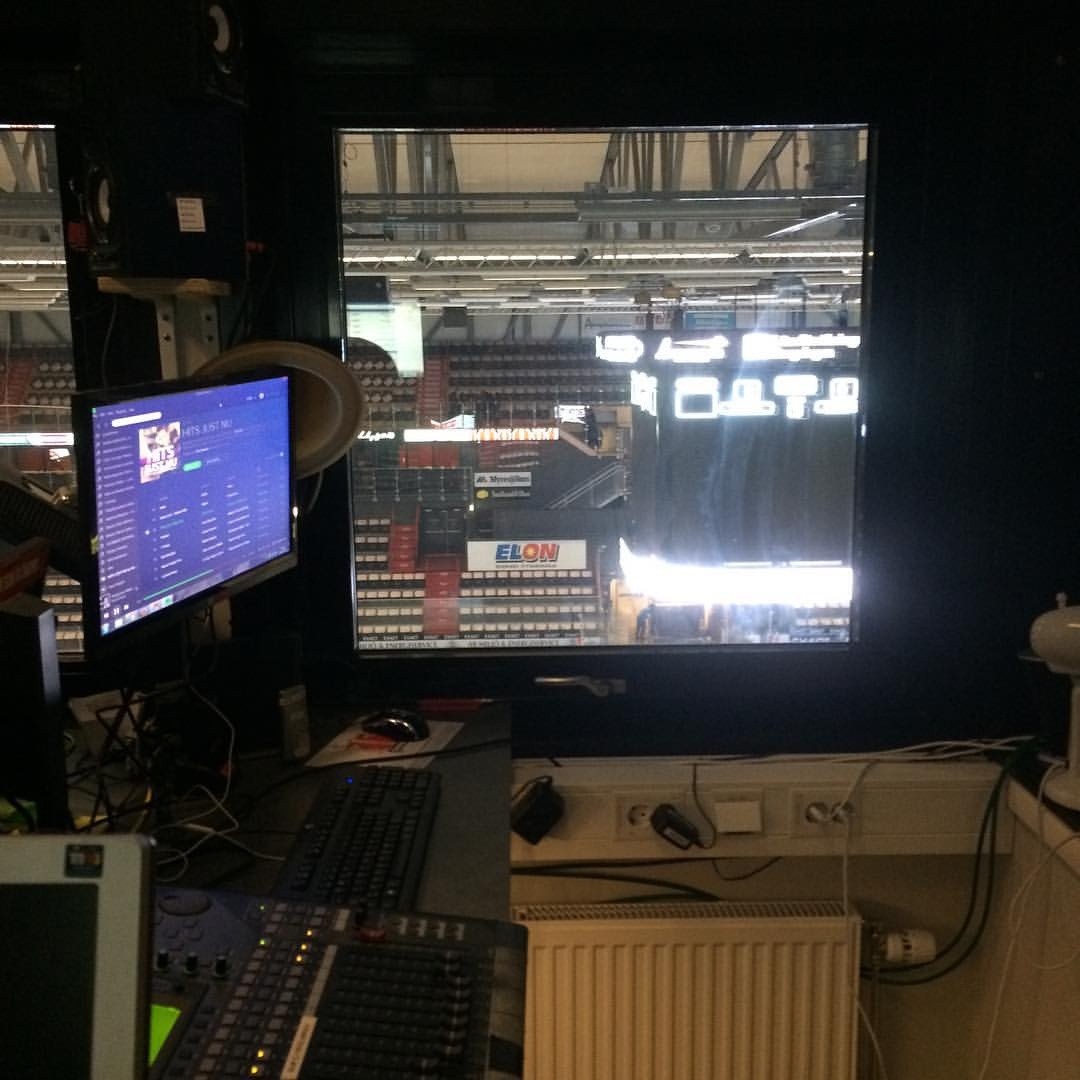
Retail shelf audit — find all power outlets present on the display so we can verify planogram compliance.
[616,791,706,844]
[788,788,863,838]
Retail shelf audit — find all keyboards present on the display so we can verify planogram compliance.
[265,763,443,915]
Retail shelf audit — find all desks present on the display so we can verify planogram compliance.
[1,699,518,1080]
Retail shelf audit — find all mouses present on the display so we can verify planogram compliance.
[360,706,432,744]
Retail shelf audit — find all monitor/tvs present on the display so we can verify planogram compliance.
[1,834,159,1080]
[69,366,302,660]
